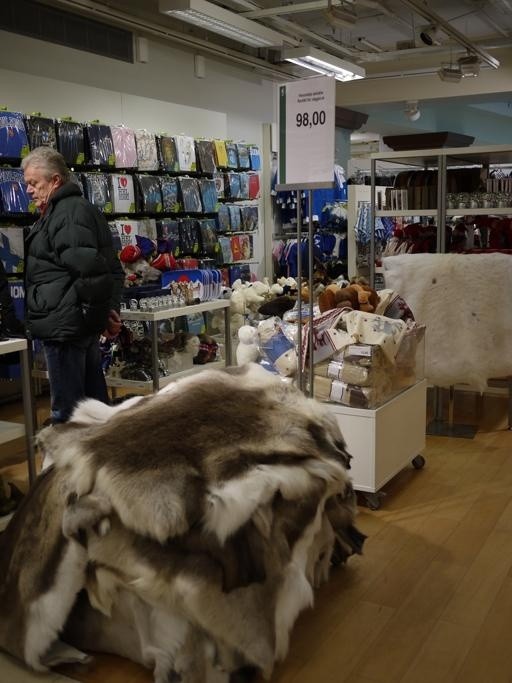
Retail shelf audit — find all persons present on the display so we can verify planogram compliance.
[19,145,123,429]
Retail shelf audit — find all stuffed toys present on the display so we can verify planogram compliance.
[218,275,381,322]
[234,326,259,367]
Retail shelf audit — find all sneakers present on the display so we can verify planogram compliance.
[193,338,217,363]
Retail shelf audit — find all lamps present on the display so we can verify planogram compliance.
[281,46,366,82]
[158,0,284,48]
